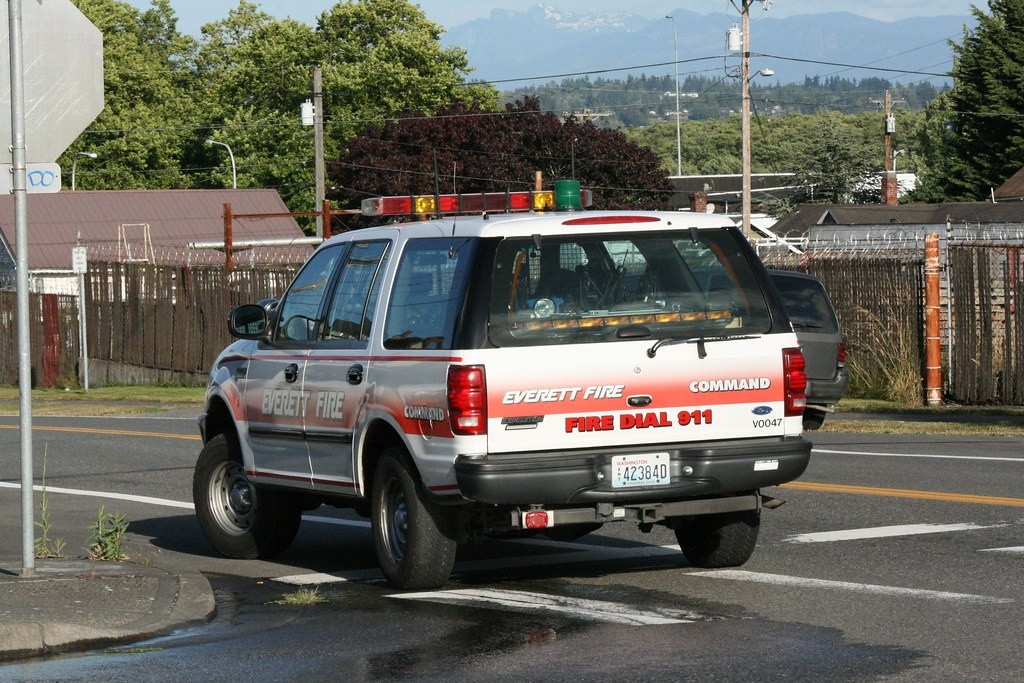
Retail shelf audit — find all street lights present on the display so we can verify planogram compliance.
[205,138,237,189]
[891,148,908,171]
[664,15,682,175]
[739,67,775,240]
[571,138,579,180]
[70,151,98,191]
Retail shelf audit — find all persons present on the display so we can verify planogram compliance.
[544,268,582,313]
[789,285,822,320]
[639,261,673,300]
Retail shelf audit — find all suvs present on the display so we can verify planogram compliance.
[624,266,850,431]
[192,176,814,594]
